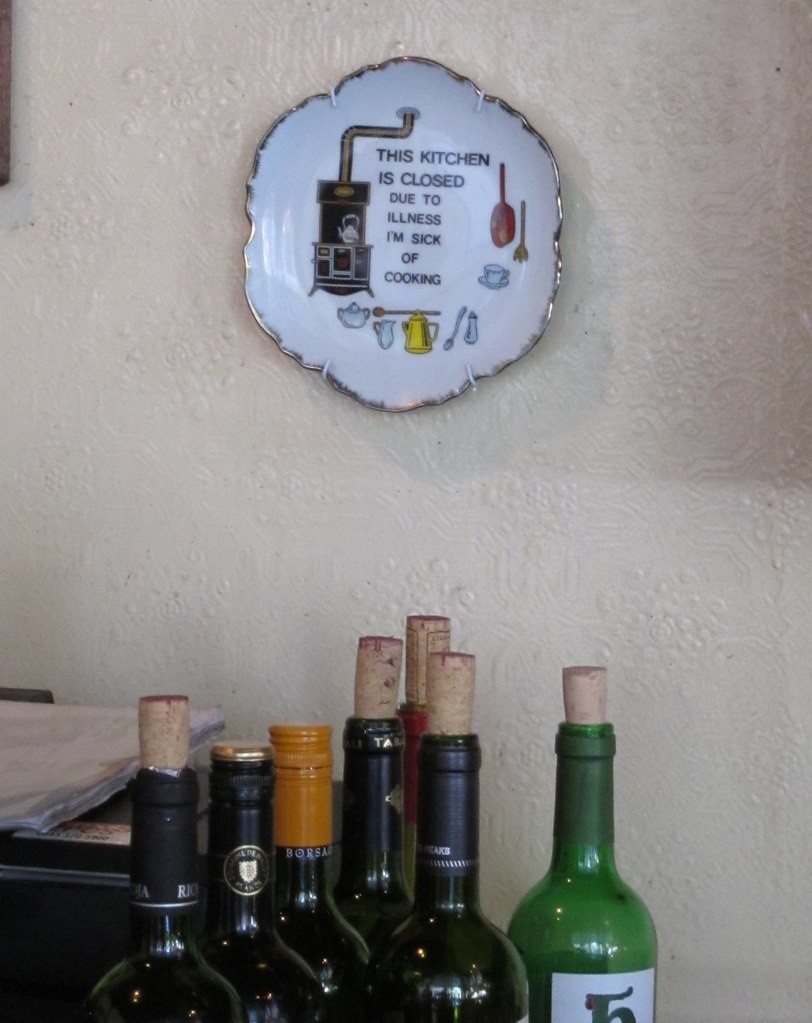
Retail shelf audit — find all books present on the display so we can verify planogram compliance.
[0,698,226,833]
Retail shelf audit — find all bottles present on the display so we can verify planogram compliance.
[83,694,246,1022]
[505,665,660,1022]
[332,636,413,954]
[401,613,454,903]
[267,726,369,1021]
[380,656,529,1022]
[199,742,322,1021]
